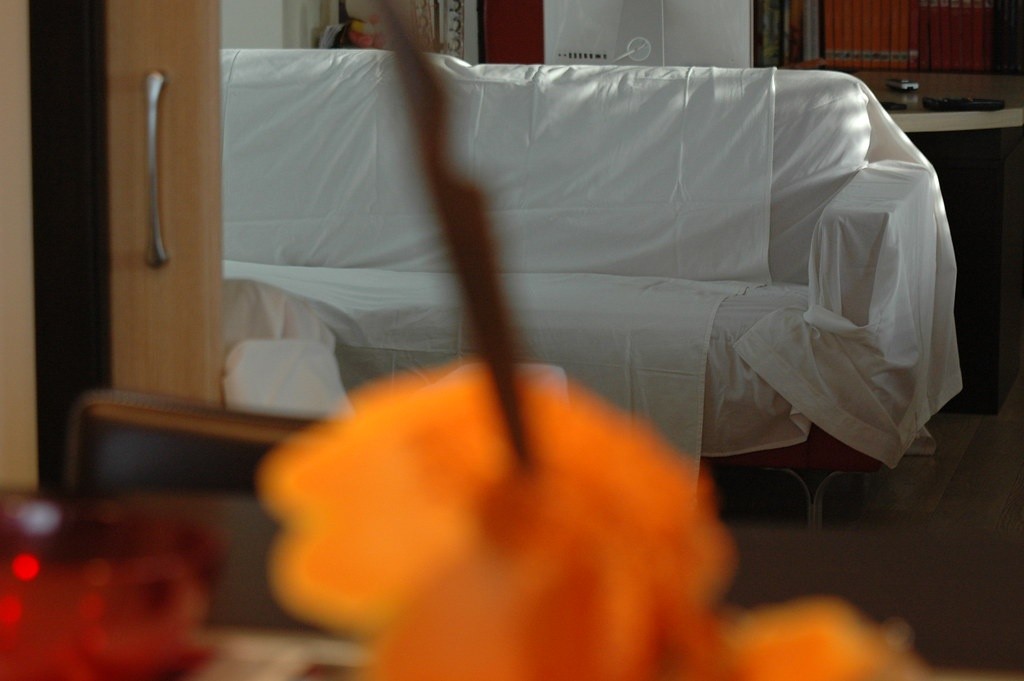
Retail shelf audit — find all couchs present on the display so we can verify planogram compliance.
[218,50,965,529]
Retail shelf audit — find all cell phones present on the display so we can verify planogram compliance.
[885,78,919,91]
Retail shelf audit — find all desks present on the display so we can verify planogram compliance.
[858,72,1024,413]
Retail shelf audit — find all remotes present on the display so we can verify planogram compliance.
[923,97,1005,112]
